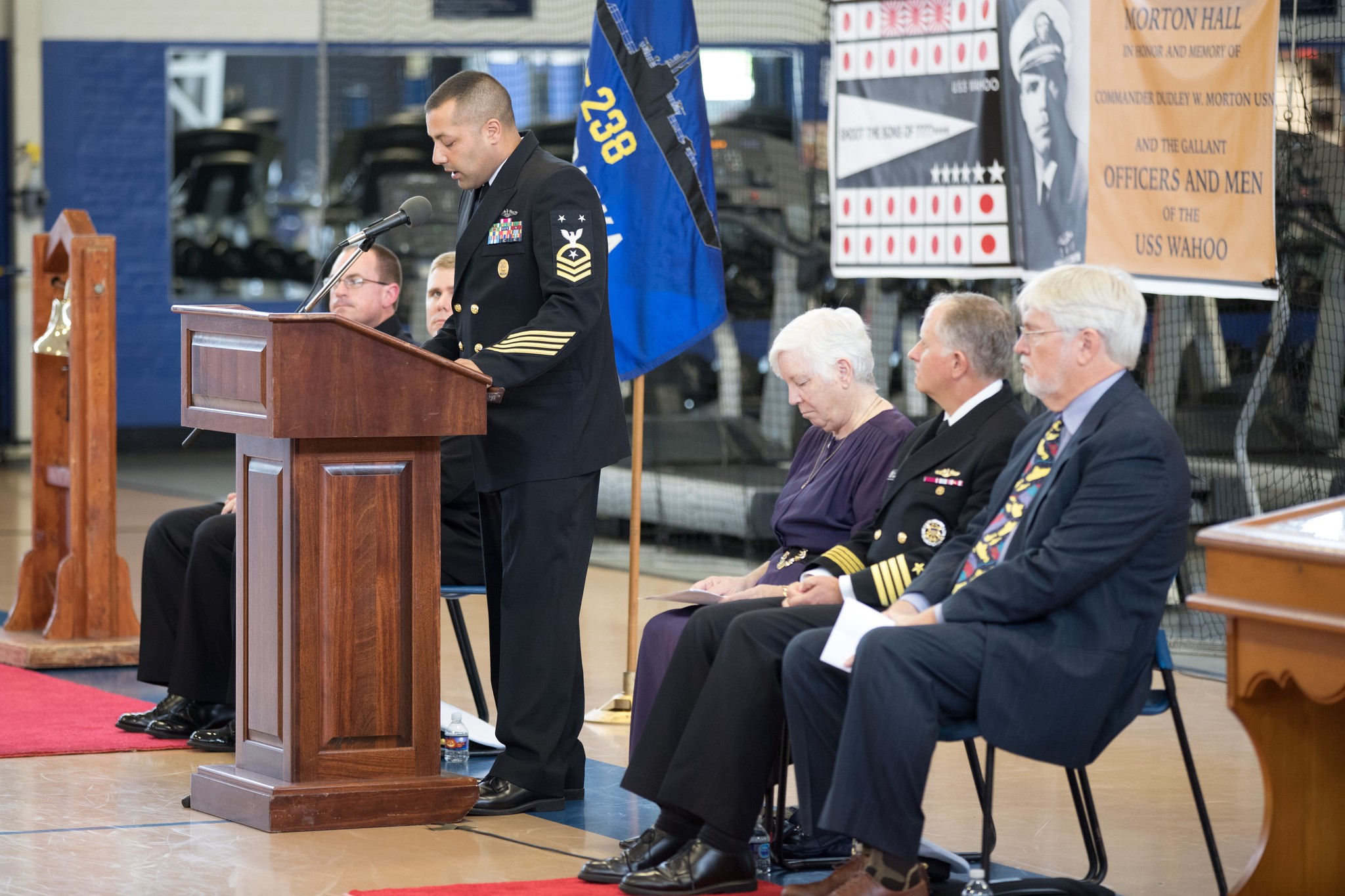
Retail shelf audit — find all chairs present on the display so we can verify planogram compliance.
[438,584,491,723]
[761,629,1233,896]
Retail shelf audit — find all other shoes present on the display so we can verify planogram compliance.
[781,832,852,866]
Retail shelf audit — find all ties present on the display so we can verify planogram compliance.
[478,183,489,203]
[934,421,951,436]
[951,417,1065,594]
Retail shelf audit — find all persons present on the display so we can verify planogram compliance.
[575,289,1030,896]
[113,243,489,752]
[780,263,1193,896]
[413,70,631,816]
[1011,0,1089,273]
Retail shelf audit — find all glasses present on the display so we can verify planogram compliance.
[323,276,391,291]
[1020,325,1093,344]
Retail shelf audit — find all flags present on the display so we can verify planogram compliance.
[571,0,728,382]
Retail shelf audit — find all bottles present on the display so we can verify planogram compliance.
[961,868,993,896]
[748,815,771,874]
[444,712,470,763]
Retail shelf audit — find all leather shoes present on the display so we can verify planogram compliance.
[468,776,566,816]
[188,719,237,750]
[114,693,184,732]
[563,780,584,801]
[144,702,212,739]
[578,825,685,883]
[619,837,757,896]
[781,852,930,896]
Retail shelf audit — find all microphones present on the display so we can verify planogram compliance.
[338,195,433,249]
